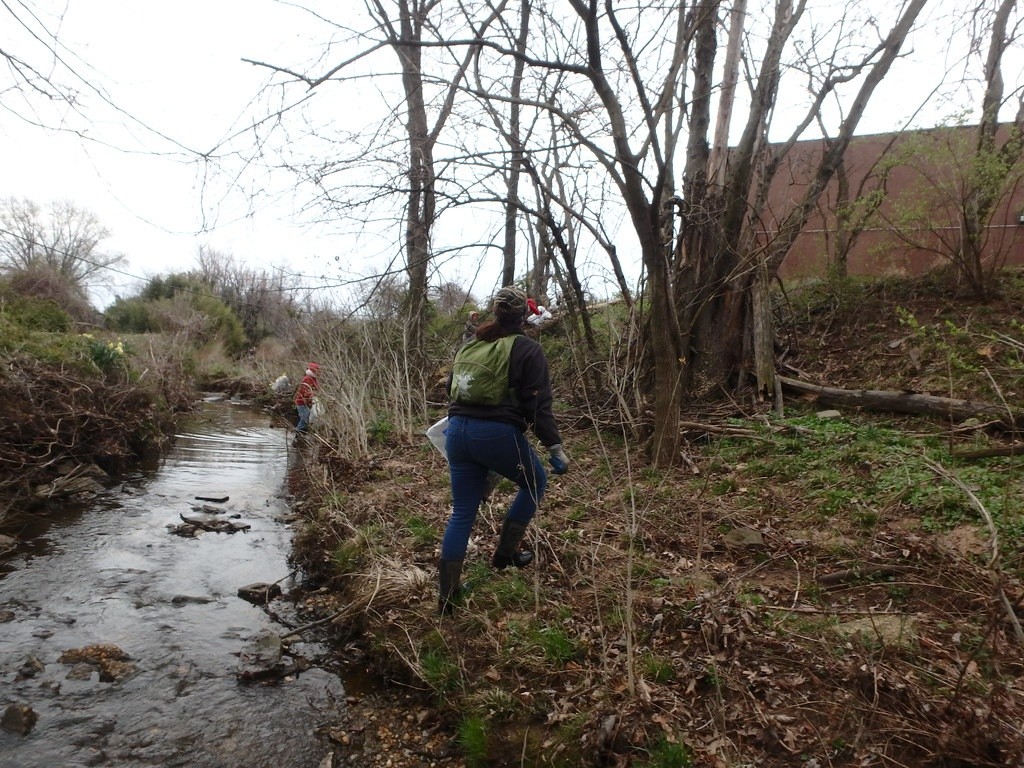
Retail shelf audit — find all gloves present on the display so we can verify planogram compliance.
[548,444,570,475]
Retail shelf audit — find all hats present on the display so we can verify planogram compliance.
[309,362,319,374]
[494,286,528,323]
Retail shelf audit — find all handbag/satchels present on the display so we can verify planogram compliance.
[308,397,325,423]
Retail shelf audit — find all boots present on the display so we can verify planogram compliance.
[292,432,305,447]
[438,558,473,614]
[492,515,532,569]
